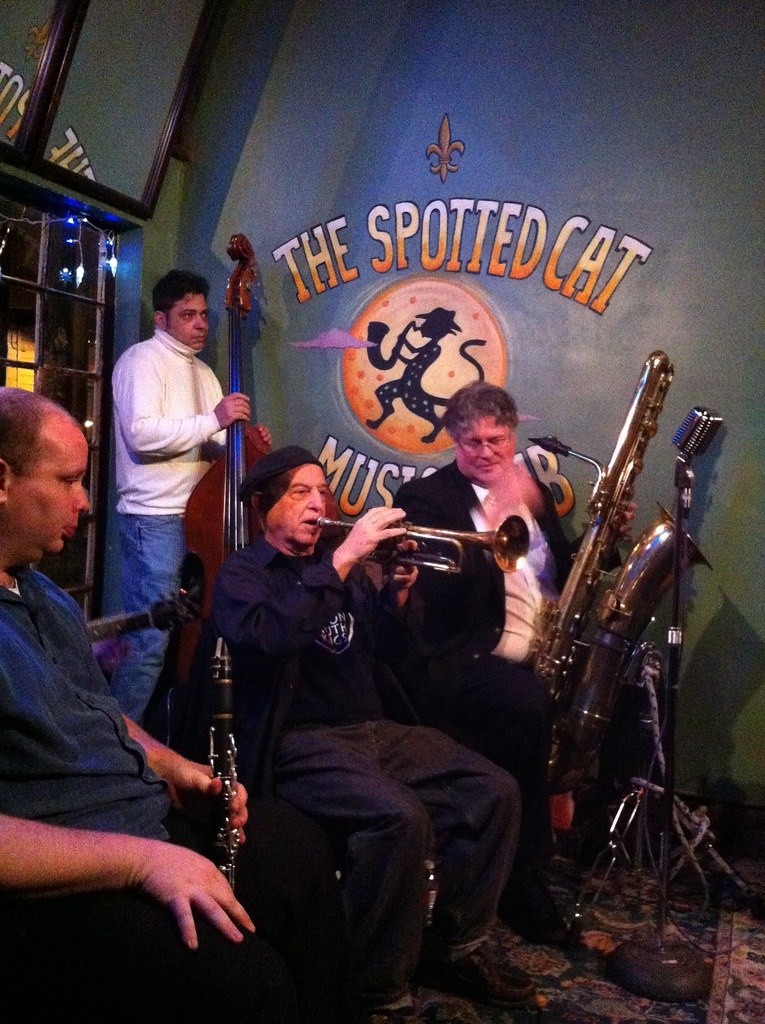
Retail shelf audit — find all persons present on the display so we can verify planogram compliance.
[194,445,536,1024]
[105,269,272,725]
[0,388,360,1024]
[383,381,637,950]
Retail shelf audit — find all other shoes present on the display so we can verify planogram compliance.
[363,990,420,1024]
[498,851,568,942]
[414,927,535,1008]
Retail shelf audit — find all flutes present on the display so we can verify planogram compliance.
[203,633,244,889]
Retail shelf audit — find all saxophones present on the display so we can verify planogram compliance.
[531,347,695,786]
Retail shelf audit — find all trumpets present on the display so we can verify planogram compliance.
[314,512,534,578]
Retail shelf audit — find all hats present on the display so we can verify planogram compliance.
[237,445,323,501]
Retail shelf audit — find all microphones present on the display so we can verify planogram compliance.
[670,405,724,457]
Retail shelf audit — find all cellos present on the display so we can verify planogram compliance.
[169,232,338,700]
